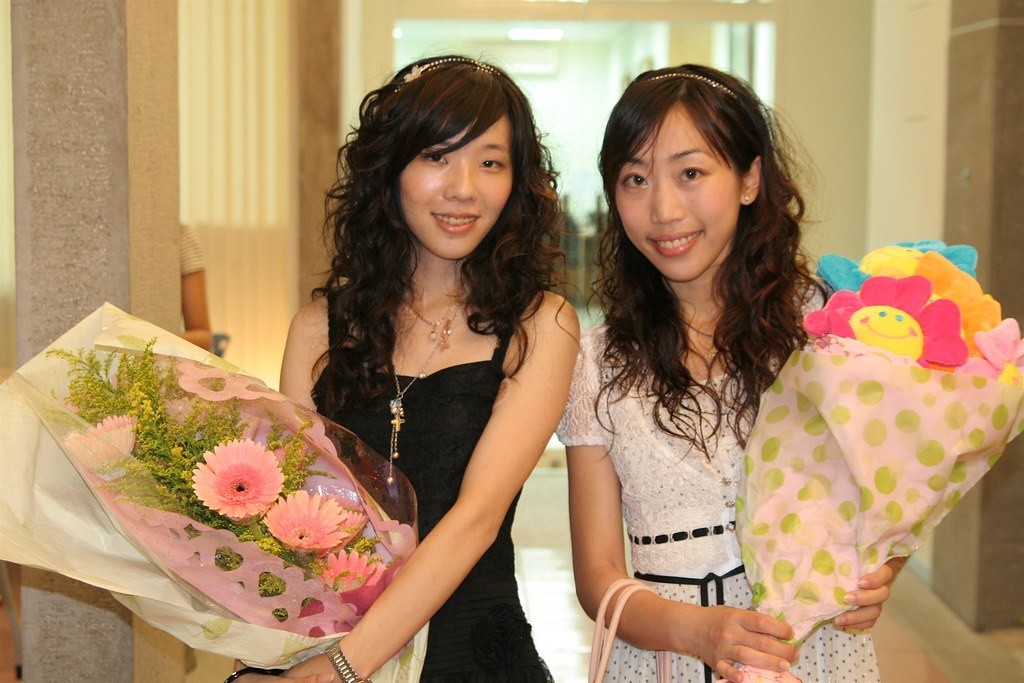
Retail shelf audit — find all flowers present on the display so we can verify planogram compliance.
[0,301,431,683]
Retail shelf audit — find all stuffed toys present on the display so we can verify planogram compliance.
[804,239,1024,375]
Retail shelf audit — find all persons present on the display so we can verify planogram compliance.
[556,63,909,683]
[224,55,581,683]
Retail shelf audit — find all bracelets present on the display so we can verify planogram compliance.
[224,667,269,683]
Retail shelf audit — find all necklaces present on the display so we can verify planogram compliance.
[687,334,720,360]
[396,295,465,341]
[383,302,467,485]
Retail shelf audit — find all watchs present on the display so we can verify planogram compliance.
[325,640,372,683]
